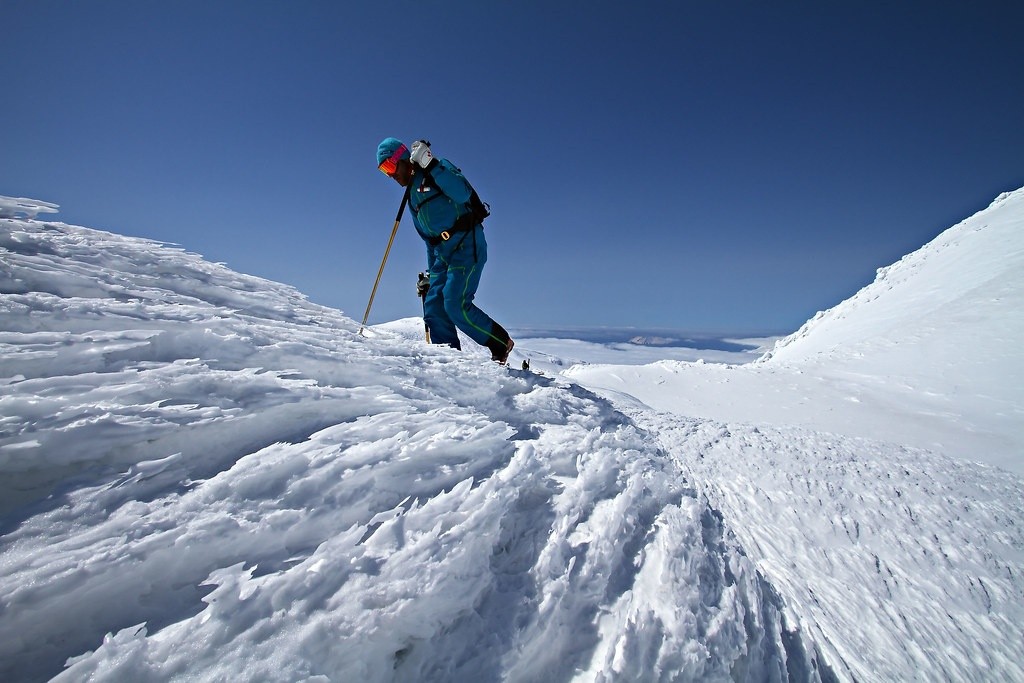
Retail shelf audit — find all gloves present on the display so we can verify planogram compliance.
[409,141,433,168]
[417,273,430,297]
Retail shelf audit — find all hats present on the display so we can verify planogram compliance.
[376,138,410,165]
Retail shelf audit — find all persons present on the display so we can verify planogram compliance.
[377,137,514,364]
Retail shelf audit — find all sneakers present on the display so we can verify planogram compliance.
[492,338,514,364]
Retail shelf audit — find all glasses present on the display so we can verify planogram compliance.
[379,144,407,177]
